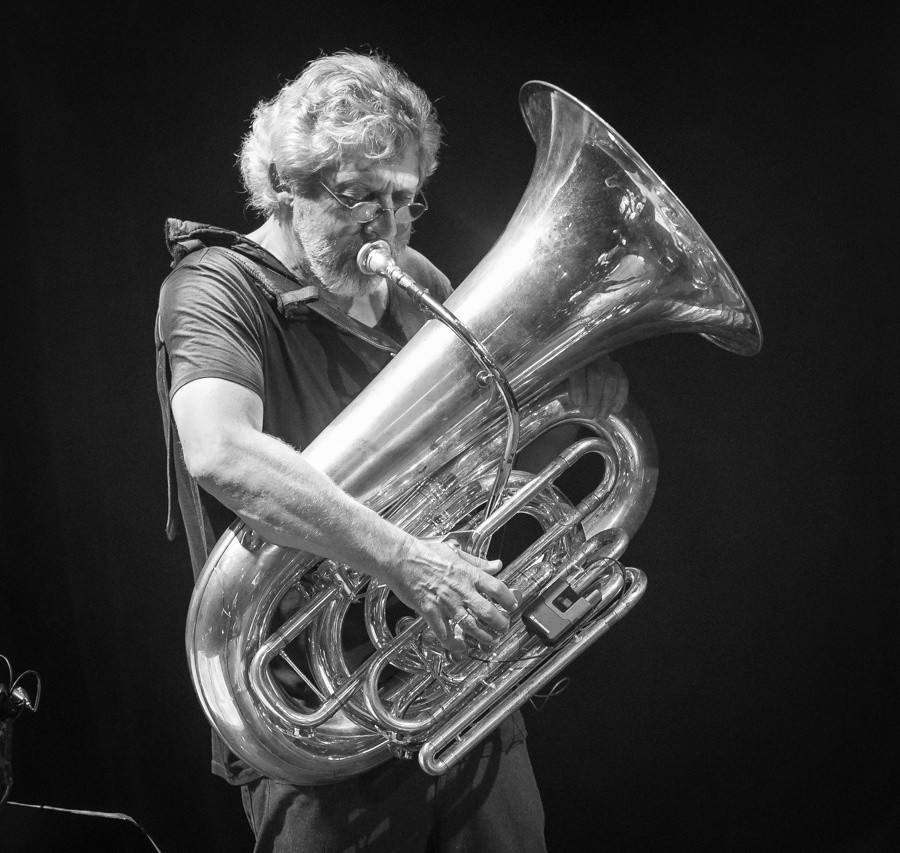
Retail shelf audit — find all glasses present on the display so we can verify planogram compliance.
[316,180,429,224]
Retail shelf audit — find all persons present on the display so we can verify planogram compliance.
[154,52,547,853]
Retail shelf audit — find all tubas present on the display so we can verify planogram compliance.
[183,79,766,787]
